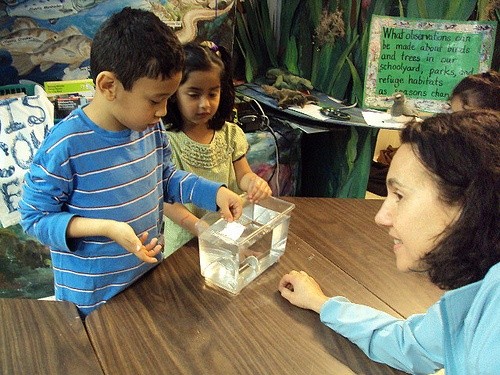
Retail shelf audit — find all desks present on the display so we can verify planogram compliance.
[85,196,448,375]
[0,298,104,375]
[236,83,424,199]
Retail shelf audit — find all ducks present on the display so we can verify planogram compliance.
[381,93,434,123]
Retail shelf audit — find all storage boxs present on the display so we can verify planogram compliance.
[45,79,94,93]
[195,191,296,295]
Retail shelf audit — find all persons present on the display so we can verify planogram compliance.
[449,70,500,113]
[16,7,245,320]
[278,109,500,375]
[161,42,272,261]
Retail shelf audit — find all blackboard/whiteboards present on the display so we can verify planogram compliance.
[362,14,497,118]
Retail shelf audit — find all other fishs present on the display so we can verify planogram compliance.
[205,255,260,276]
